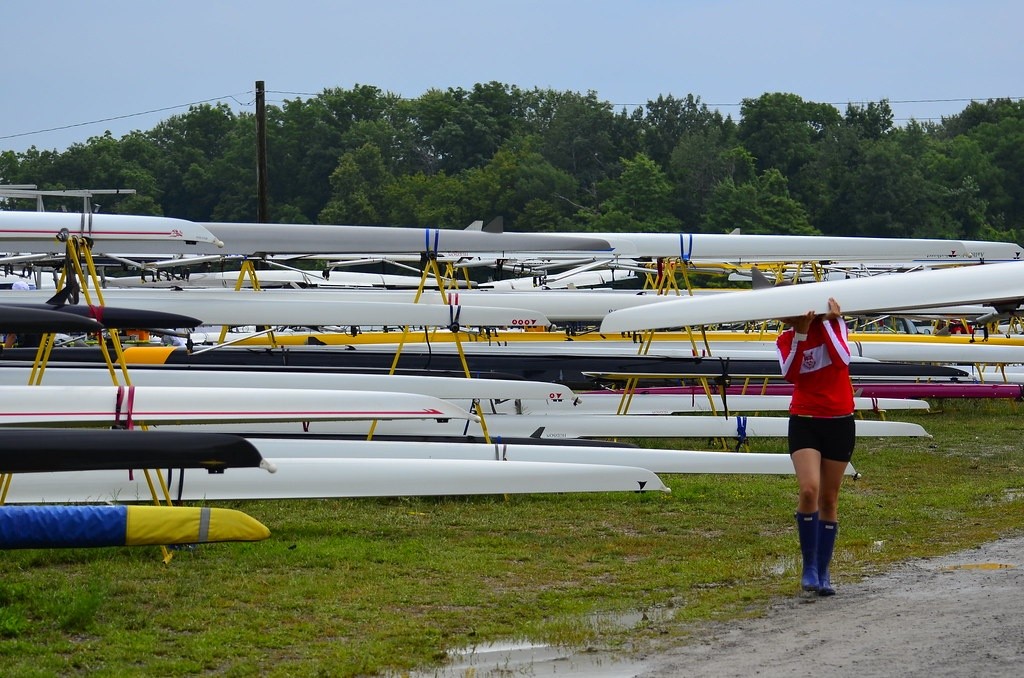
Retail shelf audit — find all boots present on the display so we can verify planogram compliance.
[796,510,820,591]
[819,520,839,596]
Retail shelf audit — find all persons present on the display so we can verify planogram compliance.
[776,297,856,594]
[5,281,43,348]
[151,329,185,346]
[936,320,975,366]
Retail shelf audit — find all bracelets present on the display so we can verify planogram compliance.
[795,332,807,341]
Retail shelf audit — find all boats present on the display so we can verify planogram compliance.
[1,186,1023,546]
